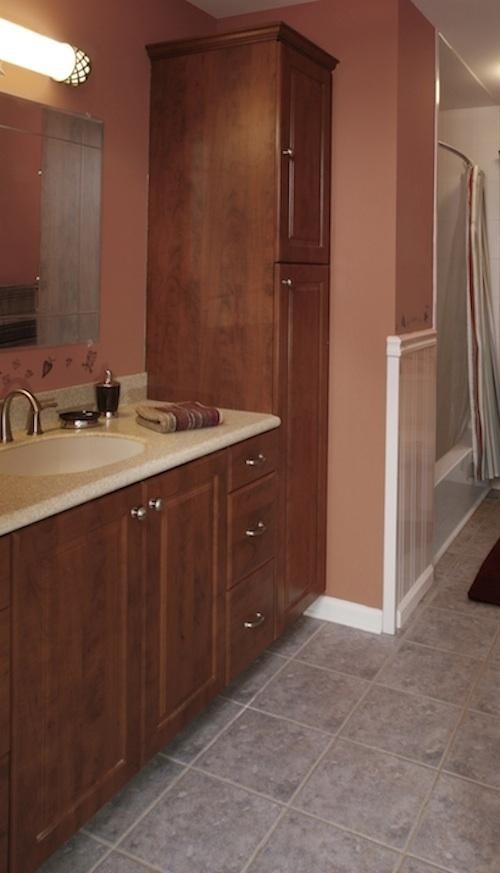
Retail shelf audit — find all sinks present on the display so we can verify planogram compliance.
[0,432,151,476]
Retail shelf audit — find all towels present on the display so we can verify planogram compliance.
[134,395,222,434]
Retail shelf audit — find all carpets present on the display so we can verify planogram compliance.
[467,540,499,606]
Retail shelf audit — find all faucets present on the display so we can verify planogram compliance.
[0,390,44,442]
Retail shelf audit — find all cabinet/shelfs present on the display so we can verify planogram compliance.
[2,450,232,870]
[139,22,335,626]
[212,427,281,694]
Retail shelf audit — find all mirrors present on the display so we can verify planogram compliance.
[1,94,104,350]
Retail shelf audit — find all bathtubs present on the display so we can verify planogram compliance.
[432,426,473,487]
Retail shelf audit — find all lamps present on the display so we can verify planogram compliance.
[1,18,92,88]
